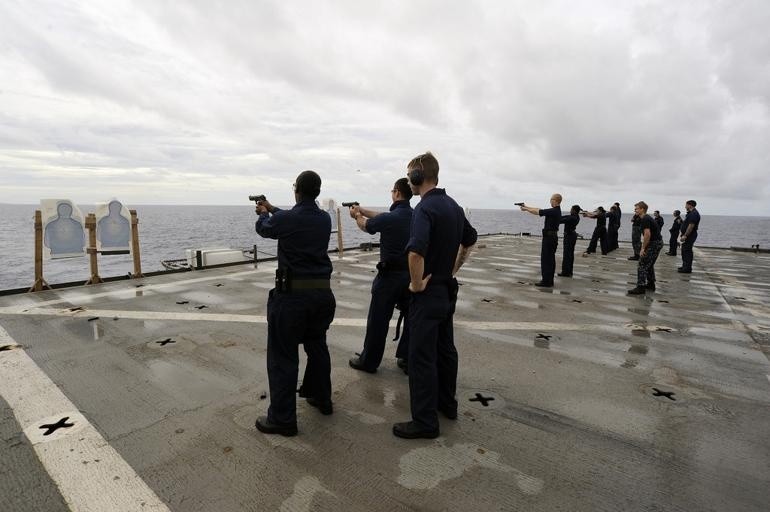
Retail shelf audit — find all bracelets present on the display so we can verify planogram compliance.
[355,212,361,220]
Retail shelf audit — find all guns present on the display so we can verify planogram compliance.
[515,203,524,206]
[342,202,359,207]
[249,195,266,204]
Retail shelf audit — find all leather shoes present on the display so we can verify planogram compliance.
[628,287,645,295]
[398,357,409,376]
[349,358,377,373]
[665,252,677,256]
[678,267,692,273]
[645,284,655,291]
[557,272,573,276]
[535,282,554,287]
[305,398,332,415]
[438,407,458,421]
[255,416,298,436]
[628,256,639,260]
[393,421,440,439]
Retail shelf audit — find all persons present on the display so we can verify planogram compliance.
[393,155,478,438]
[350,178,414,372]
[255,171,335,436]
[522,195,701,296]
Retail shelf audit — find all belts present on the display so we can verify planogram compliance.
[290,279,330,288]
